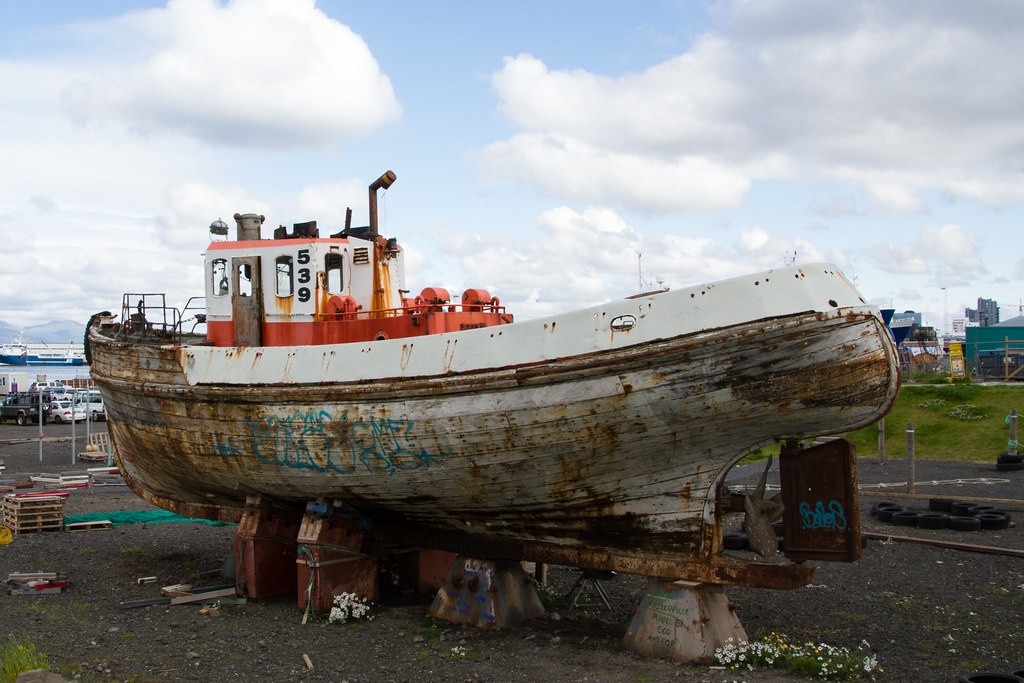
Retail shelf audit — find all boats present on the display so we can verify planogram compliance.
[84,170,902,589]
[0,331,87,366]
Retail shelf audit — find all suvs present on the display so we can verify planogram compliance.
[0,394,53,426]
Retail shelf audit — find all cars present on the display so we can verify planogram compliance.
[29,382,108,424]
